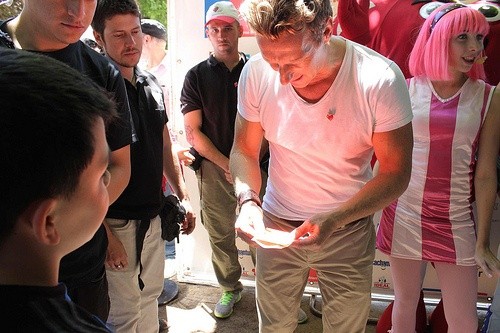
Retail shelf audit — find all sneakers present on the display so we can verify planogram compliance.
[214,290,241,318]
[298,309,307,323]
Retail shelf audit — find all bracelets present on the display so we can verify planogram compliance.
[239,190,261,209]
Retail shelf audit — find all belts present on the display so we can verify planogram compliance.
[284,217,366,232]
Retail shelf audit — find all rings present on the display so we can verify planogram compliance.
[116,264,122,268]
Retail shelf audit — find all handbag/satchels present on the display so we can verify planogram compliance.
[159,194,186,243]
[187,146,203,172]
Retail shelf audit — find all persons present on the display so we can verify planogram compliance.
[0,0,195,333]
[229,0,413,333]
[180,0,307,325]
[378,2,500,333]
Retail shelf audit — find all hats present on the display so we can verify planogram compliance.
[205,1,241,27]
[141,19,167,41]
